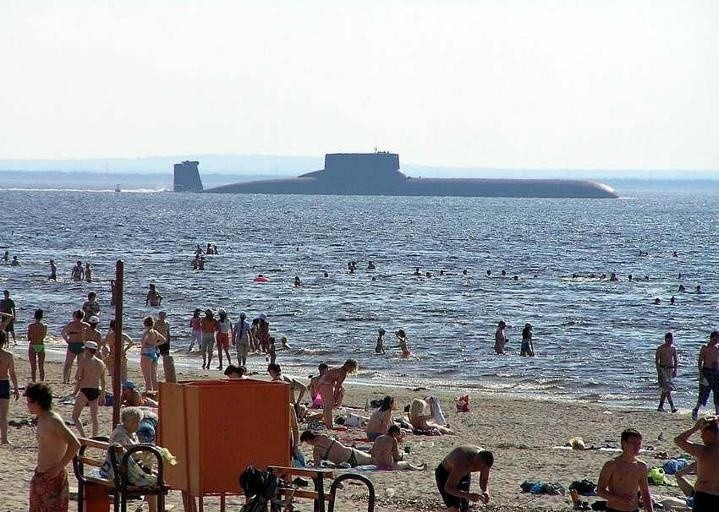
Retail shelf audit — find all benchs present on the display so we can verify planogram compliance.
[70,433,170,512]
[261,462,377,511]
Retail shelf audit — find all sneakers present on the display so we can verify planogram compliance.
[657,407,668,412]
[692,408,697,421]
[672,408,677,412]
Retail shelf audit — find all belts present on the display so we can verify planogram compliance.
[660,364,675,369]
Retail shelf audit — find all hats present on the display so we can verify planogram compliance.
[258,313,267,320]
[218,308,226,314]
[205,310,212,315]
[81,340,97,349]
[240,312,246,318]
[280,336,287,343]
[88,315,99,323]
[525,323,534,328]
